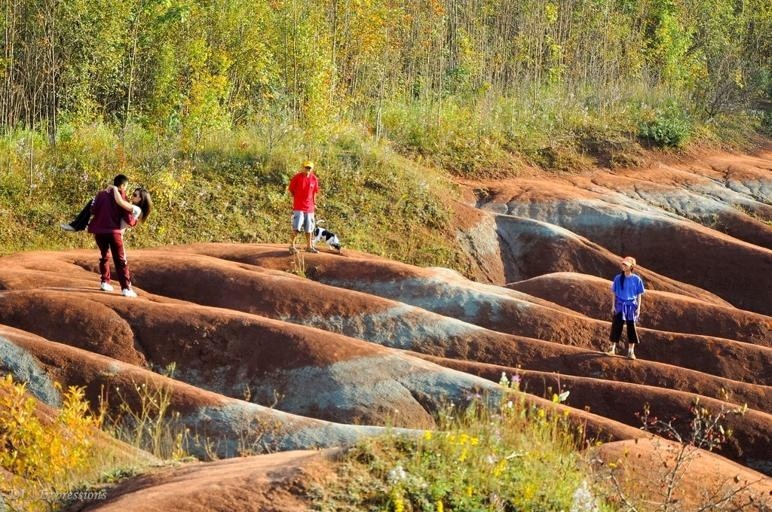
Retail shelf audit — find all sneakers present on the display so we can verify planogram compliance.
[60,224,76,230]
[288,245,319,255]
[101,282,114,291]
[122,288,138,297]
[605,350,636,360]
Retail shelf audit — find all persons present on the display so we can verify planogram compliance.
[289,161,319,255]
[60,175,152,297]
[604,256,642,360]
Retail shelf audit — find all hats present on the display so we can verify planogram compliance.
[621,256,638,268]
[304,160,315,169]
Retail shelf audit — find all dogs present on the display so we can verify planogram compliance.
[311,220,343,254]
